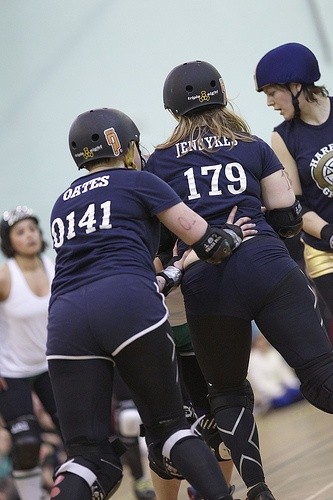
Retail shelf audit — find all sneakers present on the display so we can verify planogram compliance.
[133,479,156,500]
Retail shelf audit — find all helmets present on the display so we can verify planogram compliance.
[163,60,227,117]
[0,207,39,257]
[255,42,322,92]
[68,107,140,170]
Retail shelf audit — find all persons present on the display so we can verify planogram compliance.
[252,43,333,340]
[142,60,333,500]
[0,206,302,500]
[44,108,258,500]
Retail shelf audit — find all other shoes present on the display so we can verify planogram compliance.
[246,483,277,500]
